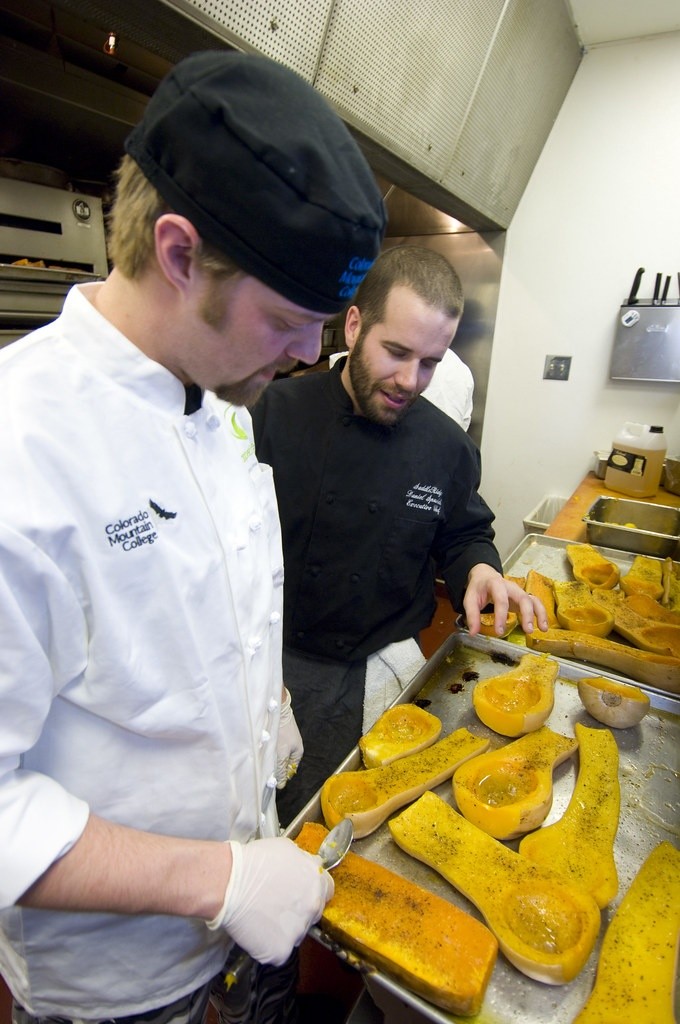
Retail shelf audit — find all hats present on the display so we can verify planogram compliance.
[124,51,386,315]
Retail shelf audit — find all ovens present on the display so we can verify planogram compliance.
[0,176,108,351]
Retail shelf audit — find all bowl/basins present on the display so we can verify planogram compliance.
[664,458,680,497]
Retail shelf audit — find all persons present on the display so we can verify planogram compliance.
[252,245,548,830]
[0,48,389,1024]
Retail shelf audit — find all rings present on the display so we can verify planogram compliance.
[528,593,532,597]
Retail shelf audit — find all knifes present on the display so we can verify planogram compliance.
[626,267,680,306]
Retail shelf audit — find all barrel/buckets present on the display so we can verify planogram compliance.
[604,422,668,498]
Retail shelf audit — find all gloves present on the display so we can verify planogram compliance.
[207,835,338,967]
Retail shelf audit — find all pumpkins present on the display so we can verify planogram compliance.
[292,542,680,1024]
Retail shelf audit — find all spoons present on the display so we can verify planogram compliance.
[227,817,355,984]
